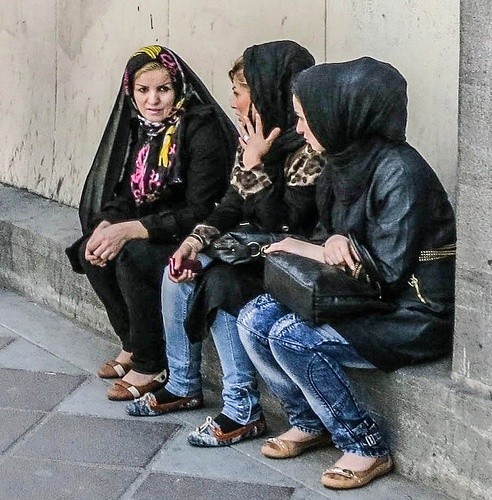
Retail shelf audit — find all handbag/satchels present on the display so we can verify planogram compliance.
[193,219,293,266]
[264,234,400,328]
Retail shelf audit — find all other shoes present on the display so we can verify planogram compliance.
[321,447,392,486]
[107,369,167,401]
[99,359,130,378]
[127,392,202,414]
[186,416,266,446]
[262,421,325,458]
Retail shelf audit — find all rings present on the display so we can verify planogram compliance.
[242,134,249,142]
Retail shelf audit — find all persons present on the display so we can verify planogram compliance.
[125,40,316,448]
[235,56,457,490]
[64,45,239,401]
